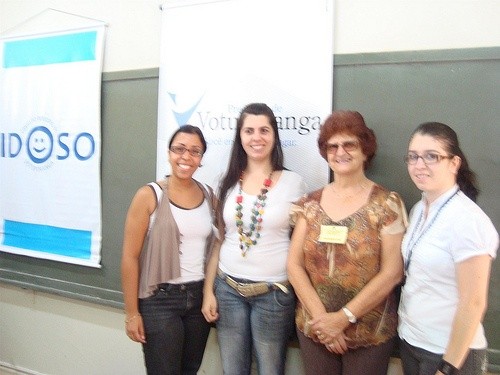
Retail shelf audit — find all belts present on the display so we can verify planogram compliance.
[158,279,204,294]
[217,267,292,298]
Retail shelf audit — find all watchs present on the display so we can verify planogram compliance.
[342,305,358,324]
[438,359,458,375]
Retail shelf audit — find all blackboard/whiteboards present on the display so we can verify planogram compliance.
[0,46,500,375]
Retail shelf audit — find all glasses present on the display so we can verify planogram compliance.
[323,141,361,154]
[169,145,203,157]
[405,151,452,165]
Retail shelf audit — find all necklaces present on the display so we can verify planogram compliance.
[405,187,459,270]
[233,169,275,257]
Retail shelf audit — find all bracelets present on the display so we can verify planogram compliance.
[125,312,140,324]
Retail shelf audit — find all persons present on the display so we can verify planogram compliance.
[200,103,310,375]
[394,121,500,375]
[121,125,219,375]
[285,109,409,375]
